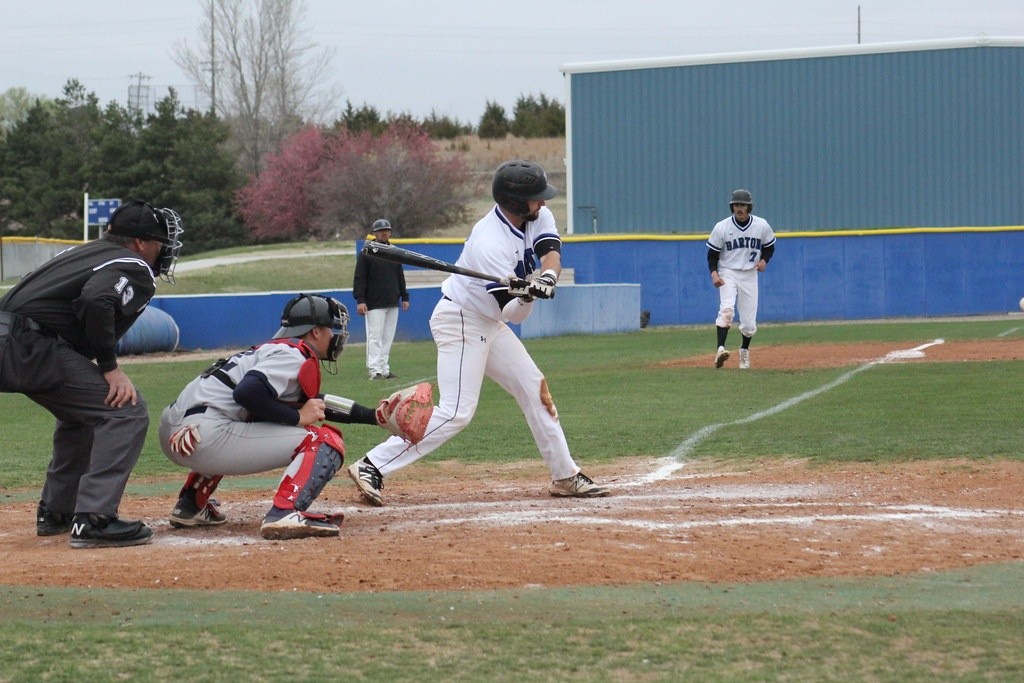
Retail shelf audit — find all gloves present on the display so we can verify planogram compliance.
[169,425,201,458]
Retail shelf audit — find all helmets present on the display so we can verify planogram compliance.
[729,189,753,214]
[492,158,557,215]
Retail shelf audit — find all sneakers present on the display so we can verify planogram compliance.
[169,499,228,527]
[260,507,339,541]
[547,473,610,499]
[69,513,155,549]
[36,500,74,536]
[348,456,385,506]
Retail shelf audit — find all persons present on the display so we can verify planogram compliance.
[347,158,612,506]
[705,189,776,370]
[0,197,185,550]
[157,293,434,541]
[352,219,410,380]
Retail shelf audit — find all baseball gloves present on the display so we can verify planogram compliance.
[376,382,436,443]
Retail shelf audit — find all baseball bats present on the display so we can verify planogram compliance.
[361,239,557,299]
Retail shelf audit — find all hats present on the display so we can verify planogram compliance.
[107,200,182,245]
[372,219,392,232]
[272,293,343,340]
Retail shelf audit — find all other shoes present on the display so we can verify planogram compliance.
[714,350,730,368]
[739,344,750,369]
